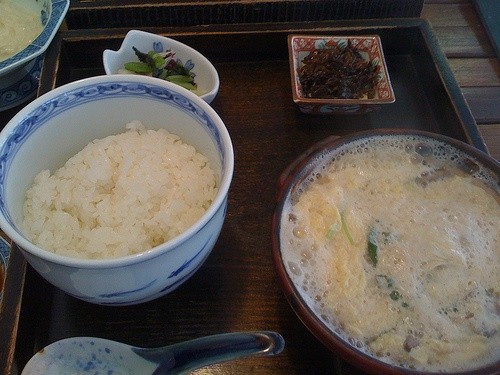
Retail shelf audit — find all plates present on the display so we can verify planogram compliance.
[103,29,220,111]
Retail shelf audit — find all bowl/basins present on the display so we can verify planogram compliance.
[1,74,234,307]
[0,0,70,88]
[273,126,500,375]
[288,32,396,114]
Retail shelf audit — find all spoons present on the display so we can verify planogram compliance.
[24,327,286,375]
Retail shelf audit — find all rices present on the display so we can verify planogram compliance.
[19,119,214,259]
